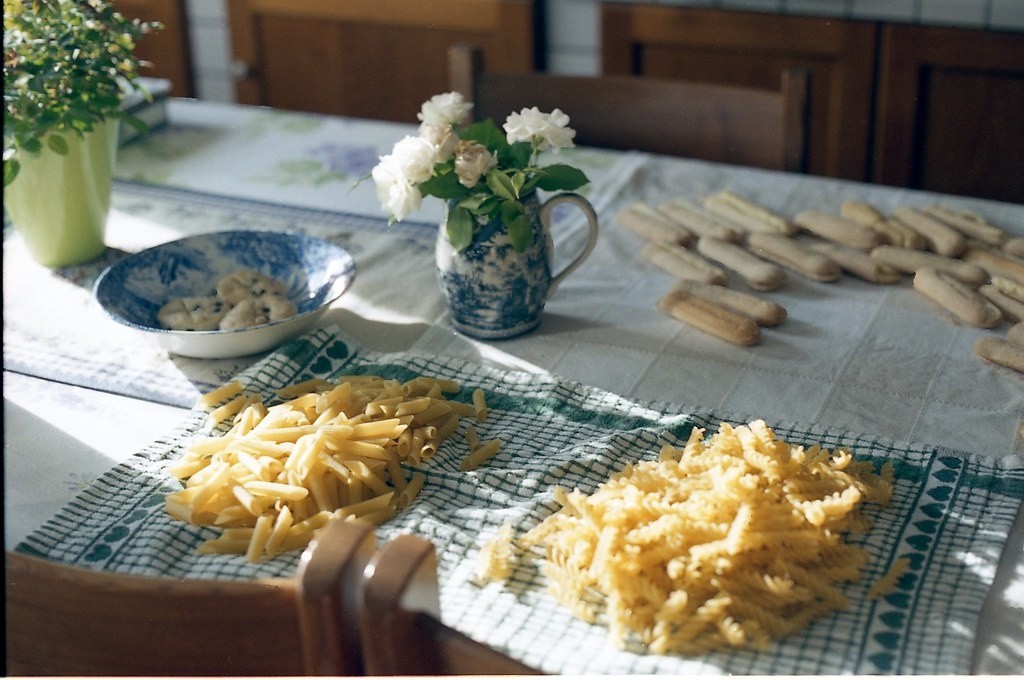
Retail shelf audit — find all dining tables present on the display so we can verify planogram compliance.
[2,96,1024,676]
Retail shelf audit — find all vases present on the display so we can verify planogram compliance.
[436,194,599,339]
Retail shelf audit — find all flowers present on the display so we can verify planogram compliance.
[370,93,593,252]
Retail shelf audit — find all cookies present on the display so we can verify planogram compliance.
[618,193,1024,368]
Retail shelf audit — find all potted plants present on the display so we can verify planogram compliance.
[1,0,167,270]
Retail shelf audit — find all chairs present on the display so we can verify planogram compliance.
[447,40,814,174]
[5,522,544,675]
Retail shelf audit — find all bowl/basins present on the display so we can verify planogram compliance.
[91,227,359,359]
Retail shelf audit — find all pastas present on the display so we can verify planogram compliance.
[161,375,500,565]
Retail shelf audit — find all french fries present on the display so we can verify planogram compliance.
[489,421,909,652]
